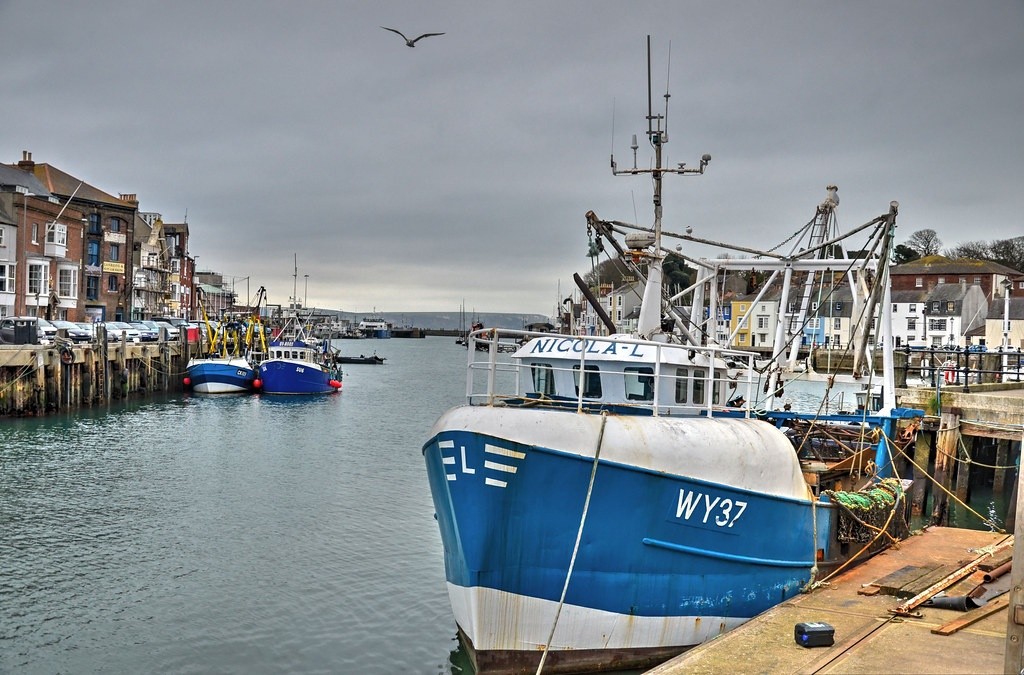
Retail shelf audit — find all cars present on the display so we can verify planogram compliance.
[0,314,272,346]
[968,345,988,352]
[994,345,1016,353]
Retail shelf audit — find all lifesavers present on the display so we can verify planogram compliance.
[944,364,956,384]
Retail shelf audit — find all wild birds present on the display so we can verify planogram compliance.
[379,26,446,48]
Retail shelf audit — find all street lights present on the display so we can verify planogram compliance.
[1000,280,1012,386]
[950,317,955,351]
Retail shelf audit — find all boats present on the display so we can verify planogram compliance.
[418,30,916,675]
[183,286,258,396]
[455,298,516,352]
[329,352,384,365]
[258,253,343,394]
